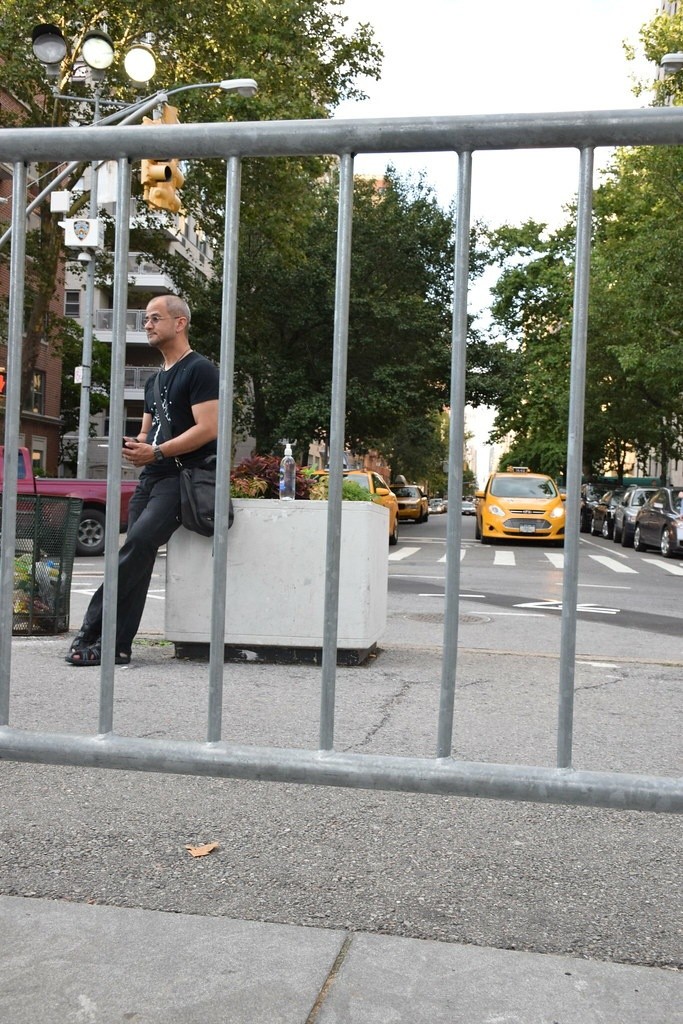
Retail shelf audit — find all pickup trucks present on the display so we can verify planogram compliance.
[0,446,139,558]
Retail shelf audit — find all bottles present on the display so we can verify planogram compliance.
[279,444,296,500]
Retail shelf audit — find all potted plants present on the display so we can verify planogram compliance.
[166,451,390,667]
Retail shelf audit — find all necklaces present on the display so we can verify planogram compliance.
[159,349,190,372]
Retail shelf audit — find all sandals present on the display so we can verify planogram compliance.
[66,642,131,665]
[70,618,100,653]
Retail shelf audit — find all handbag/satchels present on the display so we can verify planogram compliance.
[178,468,235,538]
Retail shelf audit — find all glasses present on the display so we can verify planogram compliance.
[143,316,179,325]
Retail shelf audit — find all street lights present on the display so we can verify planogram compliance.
[72,78,258,472]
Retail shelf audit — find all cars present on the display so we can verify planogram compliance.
[475,466,566,548]
[580,482,683,559]
[559,489,567,501]
[388,483,479,524]
[309,468,400,545]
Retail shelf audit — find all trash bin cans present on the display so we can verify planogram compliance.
[0,493,84,636]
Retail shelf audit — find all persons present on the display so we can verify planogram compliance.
[65,294,220,664]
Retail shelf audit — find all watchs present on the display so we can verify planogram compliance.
[154,445,164,461]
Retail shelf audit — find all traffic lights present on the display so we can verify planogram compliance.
[140,115,172,188]
[142,105,185,216]
